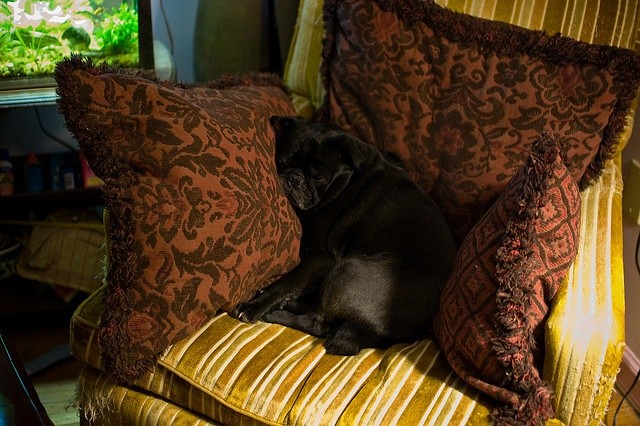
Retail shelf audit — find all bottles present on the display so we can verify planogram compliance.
[0,147,14,196]
[60,167,81,192]
[78,149,106,191]
[24,161,44,192]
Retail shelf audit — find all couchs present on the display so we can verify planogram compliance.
[68,1,640,426]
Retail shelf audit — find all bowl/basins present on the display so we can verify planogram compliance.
[0,235,25,279]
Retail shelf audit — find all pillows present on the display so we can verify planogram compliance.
[316,0,639,224]
[435,139,582,425]
[53,52,302,379]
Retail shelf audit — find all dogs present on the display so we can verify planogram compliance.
[227,115,448,354]
